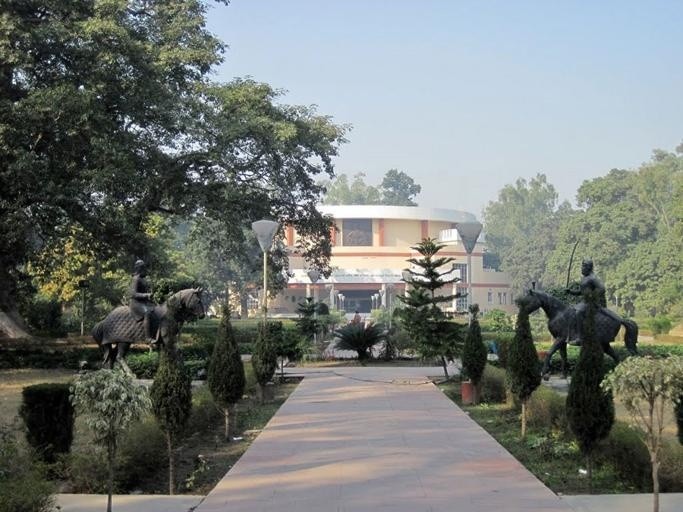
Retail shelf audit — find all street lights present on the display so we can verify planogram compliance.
[401,269,413,303]
[251,218,278,325]
[326,282,385,313]
[307,268,320,344]
[453,220,483,322]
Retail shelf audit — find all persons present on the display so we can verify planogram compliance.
[129,259,158,344]
[353,310,360,326]
[561,256,604,344]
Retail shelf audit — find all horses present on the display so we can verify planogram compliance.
[522,281,643,380]
[91,286,205,379]
